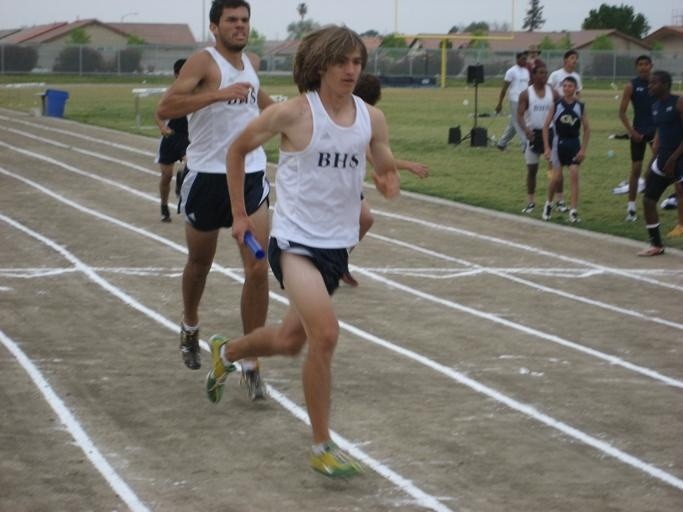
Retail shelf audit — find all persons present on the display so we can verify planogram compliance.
[154,59,189,223]
[619,56,656,221]
[205,23,403,481]
[637,70,683,257]
[341,75,428,287]
[157,1,277,402]
[494,49,590,223]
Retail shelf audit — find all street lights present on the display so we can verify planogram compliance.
[117,9,142,79]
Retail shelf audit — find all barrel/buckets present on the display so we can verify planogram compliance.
[46,88,69,118]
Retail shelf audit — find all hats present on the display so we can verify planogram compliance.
[523,44,542,56]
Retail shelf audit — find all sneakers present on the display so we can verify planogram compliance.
[624,208,639,223]
[554,199,569,214]
[339,265,359,287]
[541,200,554,222]
[239,355,269,403]
[666,223,683,239]
[567,208,582,223]
[306,438,366,483]
[179,312,202,372]
[637,246,665,257]
[521,202,536,215]
[159,204,171,223]
[204,333,236,405]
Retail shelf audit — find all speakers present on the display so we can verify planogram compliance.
[467,64,485,84]
